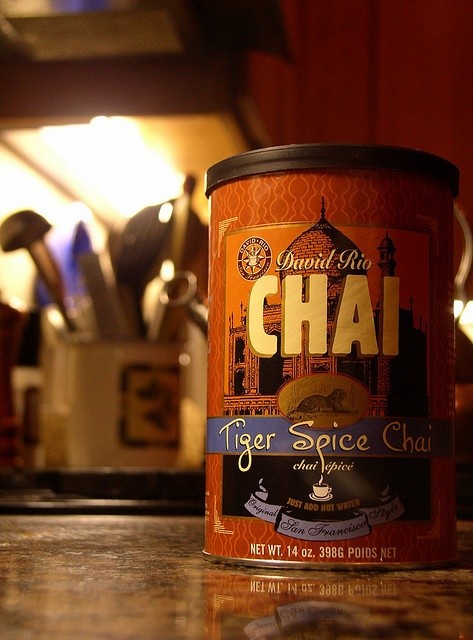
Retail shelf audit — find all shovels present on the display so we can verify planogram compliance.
[113,202,181,336]
